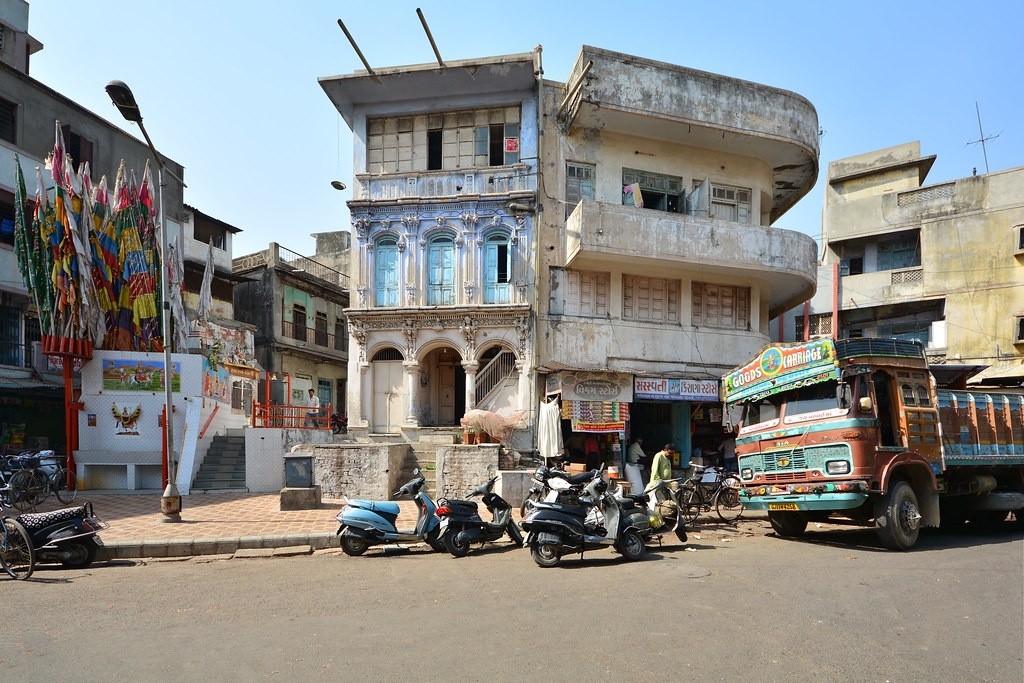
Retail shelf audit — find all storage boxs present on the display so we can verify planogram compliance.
[563,463,587,475]
[2,434,50,455]
[670,452,680,466]
[691,456,704,471]
[600,434,621,479]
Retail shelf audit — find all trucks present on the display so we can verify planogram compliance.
[719,333,1024,555]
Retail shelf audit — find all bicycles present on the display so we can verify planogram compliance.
[673,460,747,522]
[0,497,36,581]
[0,452,79,513]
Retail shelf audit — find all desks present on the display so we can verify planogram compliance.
[616,480,631,495]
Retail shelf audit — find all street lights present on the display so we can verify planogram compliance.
[104,78,181,512]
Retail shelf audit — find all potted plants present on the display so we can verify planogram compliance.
[462,426,499,445]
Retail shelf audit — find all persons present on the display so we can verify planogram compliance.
[718,438,735,470]
[628,438,651,482]
[648,444,675,511]
[304,389,319,427]
[585,438,599,470]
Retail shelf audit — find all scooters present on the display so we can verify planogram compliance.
[621,477,688,551]
[337,470,447,555]
[436,464,523,559]
[516,477,654,566]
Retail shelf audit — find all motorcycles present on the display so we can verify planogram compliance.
[13,501,111,566]
[331,414,349,434]
[518,457,620,518]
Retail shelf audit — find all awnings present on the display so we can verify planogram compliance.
[0,378,82,389]
